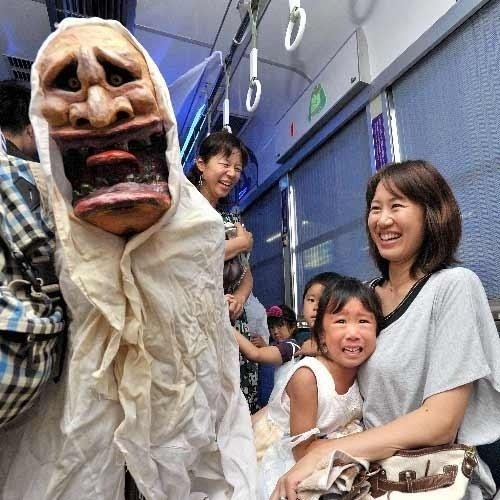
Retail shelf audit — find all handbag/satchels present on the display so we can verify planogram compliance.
[341,443,479,500]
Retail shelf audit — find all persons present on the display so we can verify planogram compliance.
[0,78,63,166]
[273,159,498,496]
[33,17,172,233]
[228,273,344,397]
[250,306,300,347]
[184,134,261,415]
[250,278,382,460]
